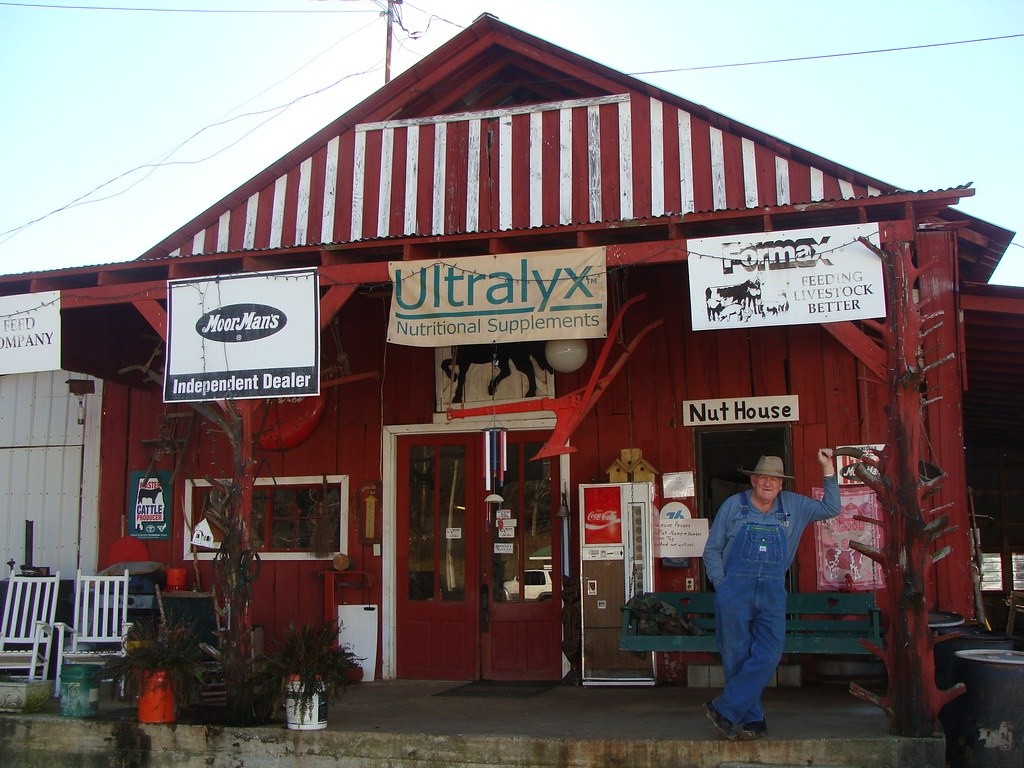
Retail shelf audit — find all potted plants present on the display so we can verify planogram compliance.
[103,613,209,723]
[249,614,366,730]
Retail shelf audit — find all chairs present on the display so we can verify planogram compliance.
[0,570,61,682]
[54,568,134,697]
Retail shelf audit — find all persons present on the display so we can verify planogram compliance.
[703,448,842,741]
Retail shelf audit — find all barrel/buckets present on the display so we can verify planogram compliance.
[954,648,1024,768]
[59,664,101,718]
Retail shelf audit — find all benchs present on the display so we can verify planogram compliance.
[620,589,882,662]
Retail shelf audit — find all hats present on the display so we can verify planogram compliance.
[740,457,796,480]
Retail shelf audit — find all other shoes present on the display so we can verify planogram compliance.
[743,721,766,738]
[704,702,739,741]
